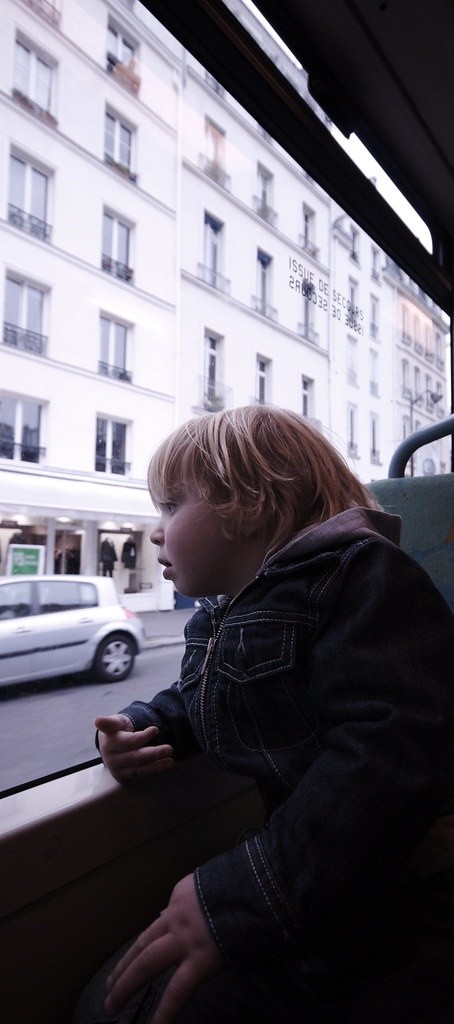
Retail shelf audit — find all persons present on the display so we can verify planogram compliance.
[99,536,118,577]
[72,411,454,1024]
[121,537,137,568]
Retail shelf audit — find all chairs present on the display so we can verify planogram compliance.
[365,413,454,613]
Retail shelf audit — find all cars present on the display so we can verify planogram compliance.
[0,575,146,685]
